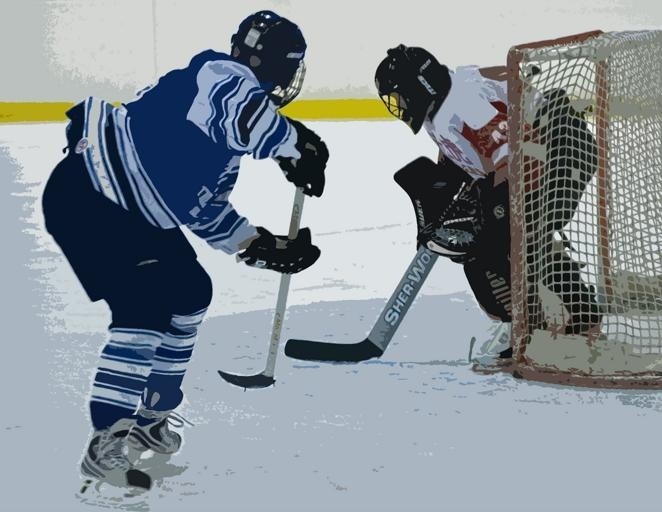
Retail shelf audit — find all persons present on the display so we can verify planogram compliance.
[374,44,603,375]
[39,9,329,489]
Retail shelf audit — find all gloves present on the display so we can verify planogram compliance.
[276,115,331,197]
[234,226,324,275]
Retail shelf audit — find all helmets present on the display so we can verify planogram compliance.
[374,43,451,135]
[227,11,307,94]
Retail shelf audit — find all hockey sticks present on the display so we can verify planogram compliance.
[284,64,543,363]
[218,143,316,389]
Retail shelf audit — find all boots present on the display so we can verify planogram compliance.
[130,392,183,455]
[74,417,155,491]
